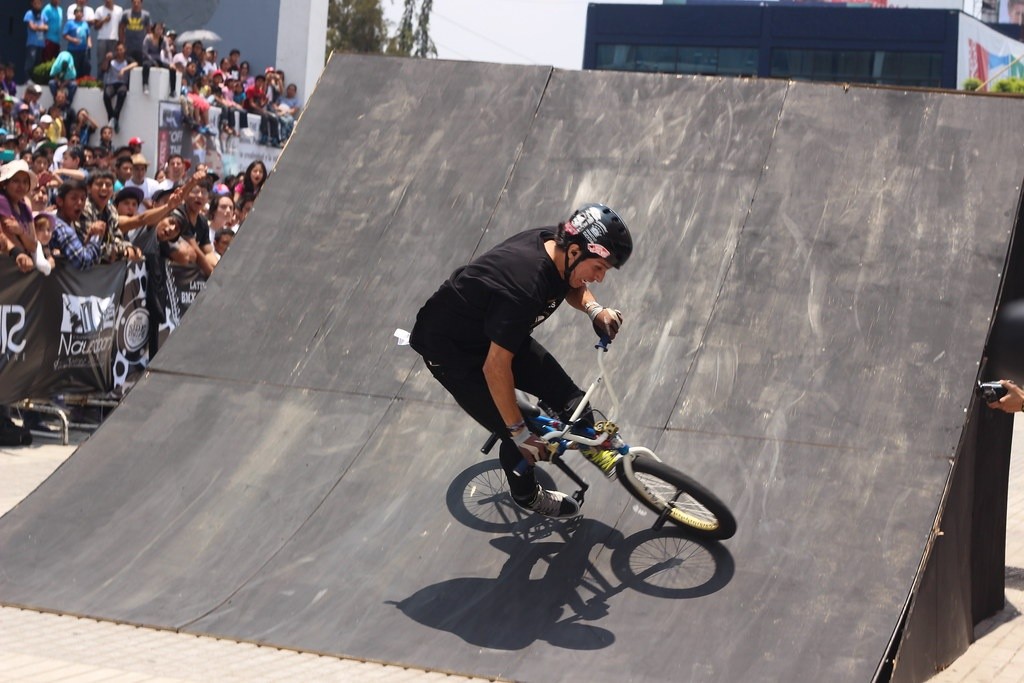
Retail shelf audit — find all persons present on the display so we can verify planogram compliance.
[409,203,634,519]
[985,380,1024,414]
[0,0,302,447]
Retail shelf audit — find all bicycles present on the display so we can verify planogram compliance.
[478,333,737,542]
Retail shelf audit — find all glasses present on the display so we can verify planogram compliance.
[136,145,142,148]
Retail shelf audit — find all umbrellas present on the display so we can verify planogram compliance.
[175,30,221,45]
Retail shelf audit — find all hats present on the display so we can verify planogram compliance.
[31,210,56,233]
[131,153,147,165]
[169,208,190,243]
[264,66,276,73]
[34,84,41,94]
[163,159,191,171]
[205,46,215,52]
[166,29,176,36]
[40,115,53,123]
[111,186,145,203]
[128,137,144,146]
[206,168,220,182]
[0,159,38,191]
[152,188,175,201]
[18,104,29,112]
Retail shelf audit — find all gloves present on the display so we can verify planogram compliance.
[509,426,551,467]
[591,306,624,344]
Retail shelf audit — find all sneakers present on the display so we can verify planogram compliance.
[579,446,625,482]
[508,480,580,519]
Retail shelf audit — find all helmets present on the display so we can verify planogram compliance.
[565,202,633,270]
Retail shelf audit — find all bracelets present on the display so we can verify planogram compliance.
[506,418,525,432]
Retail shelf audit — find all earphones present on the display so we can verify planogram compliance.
[572,251,577,256]
